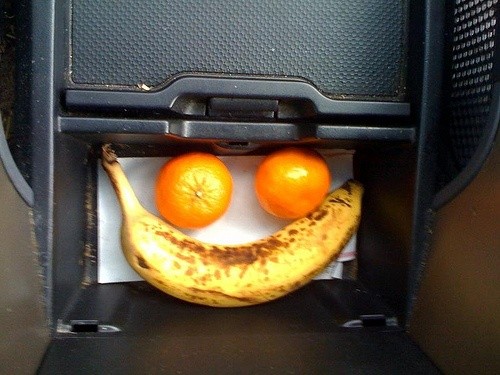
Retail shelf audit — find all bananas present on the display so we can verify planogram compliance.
[100,140,365,307]
